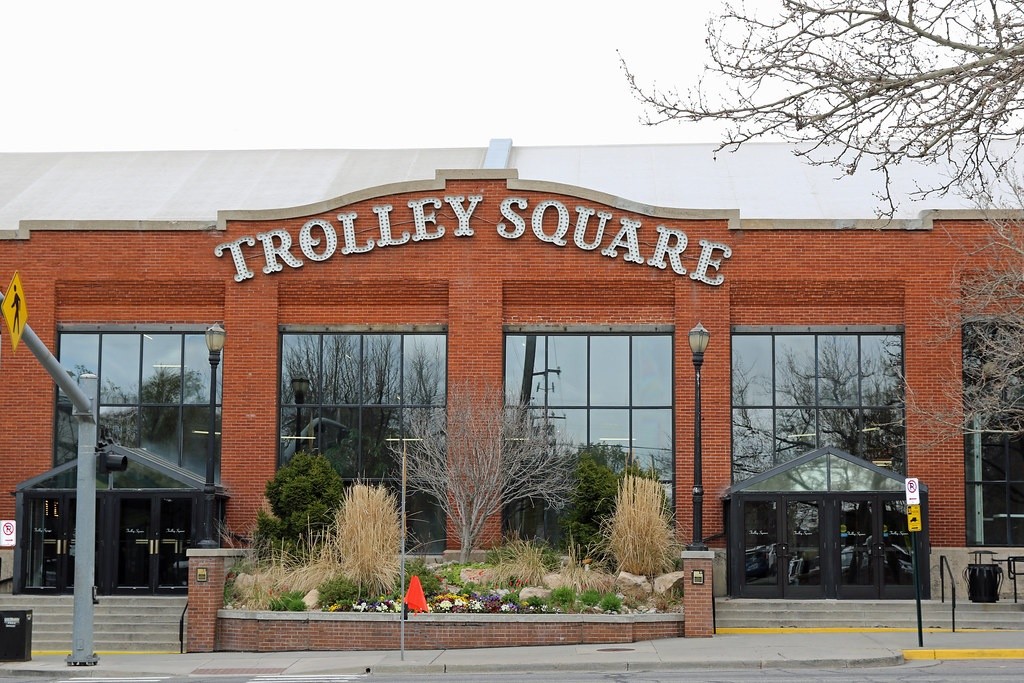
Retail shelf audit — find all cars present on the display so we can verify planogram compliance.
[745,539,913,585]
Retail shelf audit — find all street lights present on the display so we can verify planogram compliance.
[685,323,711,551]
[196,322,226,548]
[290,365,311,456]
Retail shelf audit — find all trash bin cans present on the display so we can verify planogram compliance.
[961,550,1004,603]
[0,609,33,662]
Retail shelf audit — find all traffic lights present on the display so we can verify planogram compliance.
[96,452,130,474]
